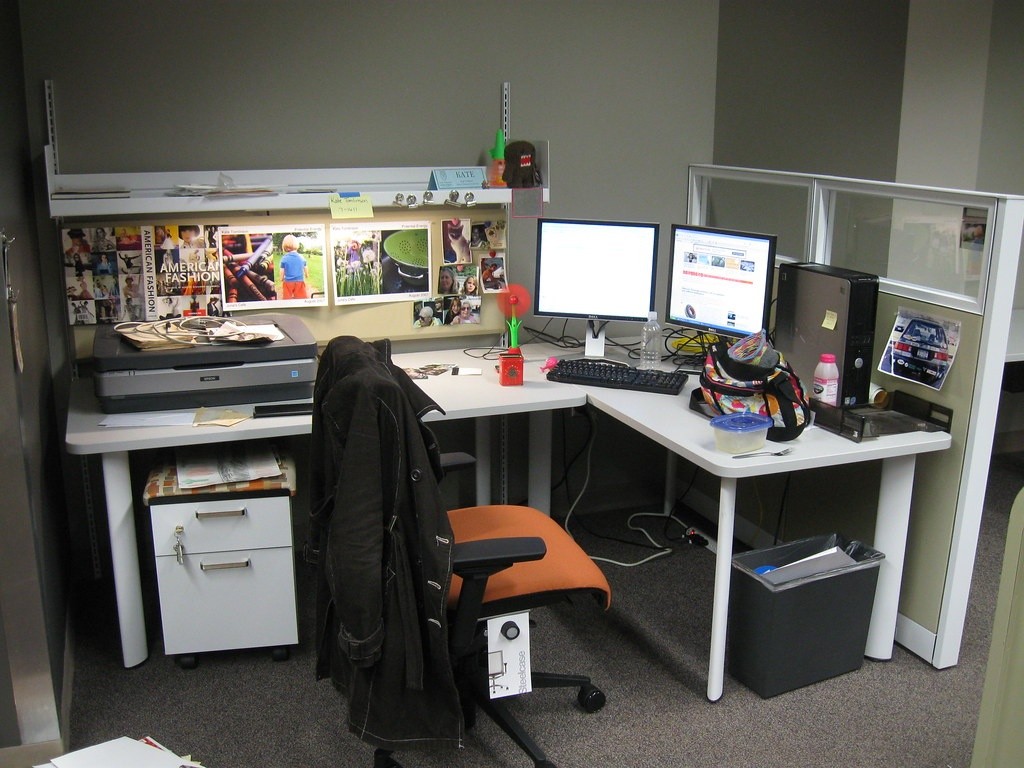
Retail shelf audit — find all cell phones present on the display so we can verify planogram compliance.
[254,403,314,418]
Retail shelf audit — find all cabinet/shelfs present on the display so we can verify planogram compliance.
[143,445,303,664]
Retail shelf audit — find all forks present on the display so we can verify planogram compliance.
[733,448,791,459]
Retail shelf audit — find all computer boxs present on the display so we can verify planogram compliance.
[773,262,880,408]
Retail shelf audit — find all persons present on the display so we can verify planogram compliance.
[280,233,310,300]
[347,239,375,274]
[154,225,220,320]
[413,228,505,327]
[62,226,144,324]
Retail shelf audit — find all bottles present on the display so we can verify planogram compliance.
[642,312,662,369]
[812,353,838,404]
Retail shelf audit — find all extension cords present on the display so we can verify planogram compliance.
[685,527,718,554]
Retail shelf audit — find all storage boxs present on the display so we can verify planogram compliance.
[709,411,774,455]
[731,533,887,696]
[498,353,526,388]
[777,262,880,408]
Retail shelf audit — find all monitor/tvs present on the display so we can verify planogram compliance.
[665,225,777,376]
[533,217,660,367]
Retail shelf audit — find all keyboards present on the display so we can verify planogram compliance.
[545,359,689,395]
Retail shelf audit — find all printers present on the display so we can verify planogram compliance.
[90,313,320,414]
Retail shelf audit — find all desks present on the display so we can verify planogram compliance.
[64,338,955,705]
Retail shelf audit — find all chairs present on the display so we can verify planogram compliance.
[314,337,613,768]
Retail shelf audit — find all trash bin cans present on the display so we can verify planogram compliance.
[730,532,886,699]
